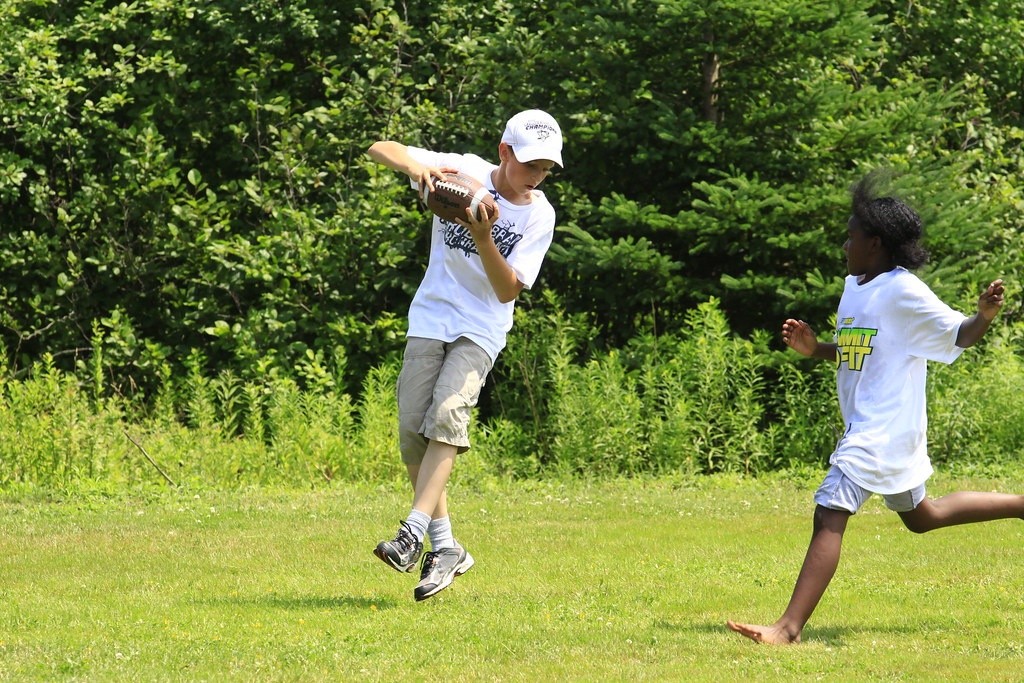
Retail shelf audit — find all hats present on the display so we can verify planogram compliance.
[501,109,564,168]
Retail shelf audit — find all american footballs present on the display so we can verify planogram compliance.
[418,172,496,223]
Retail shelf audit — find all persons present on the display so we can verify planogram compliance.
[367,109,564,600]
[726,173,1024,645]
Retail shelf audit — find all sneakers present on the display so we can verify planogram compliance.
[373,520,423,573]
[414,538,474,603]
